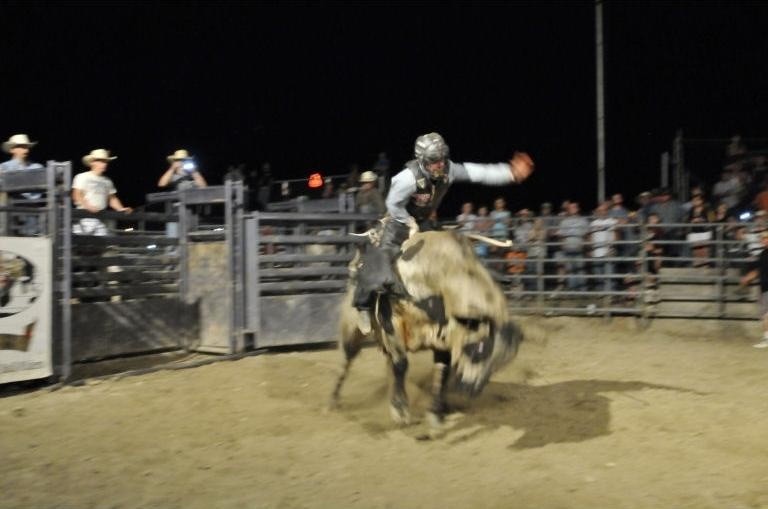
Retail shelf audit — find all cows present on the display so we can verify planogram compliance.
[325,224,548,439]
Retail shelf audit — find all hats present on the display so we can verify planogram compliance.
[358,171,379,183]
[1,134,38,153]
[82,148,118,167]
[166,150,193,164]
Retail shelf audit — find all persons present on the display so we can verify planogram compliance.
[0,132,46,236]
[157,149,211,238]
[351,128,536,316]
[70,147,133,304]
[307,157,768,348]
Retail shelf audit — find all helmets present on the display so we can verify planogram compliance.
[415,132,450,184]
